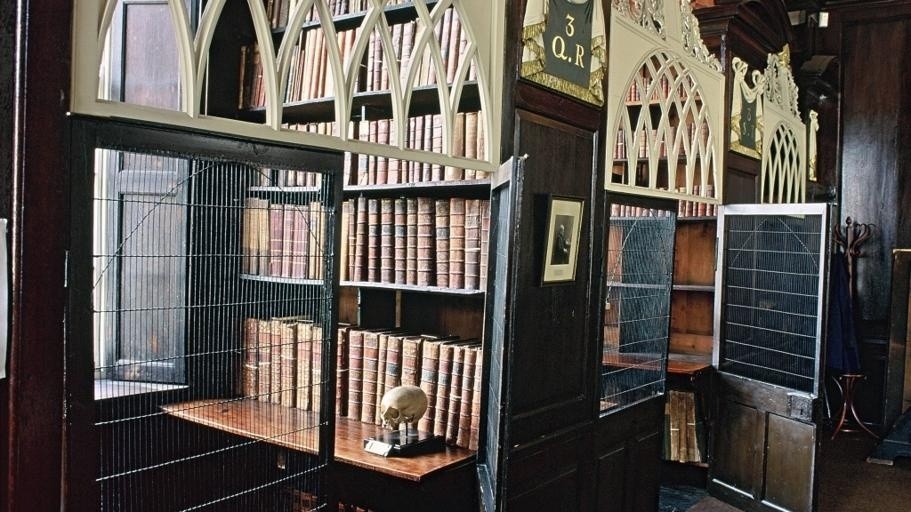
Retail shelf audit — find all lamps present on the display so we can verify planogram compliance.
[807,10,831,39]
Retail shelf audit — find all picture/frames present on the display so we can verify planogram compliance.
[539,193,586,284]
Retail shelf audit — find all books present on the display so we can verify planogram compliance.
[243,317,482,452]
[236,1,489,290]
[281,484,374,512]
[663,381,710,462]
[611,74,718,217]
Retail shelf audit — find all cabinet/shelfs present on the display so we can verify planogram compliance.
[158,1,491,511]
[601,95,720,488]
